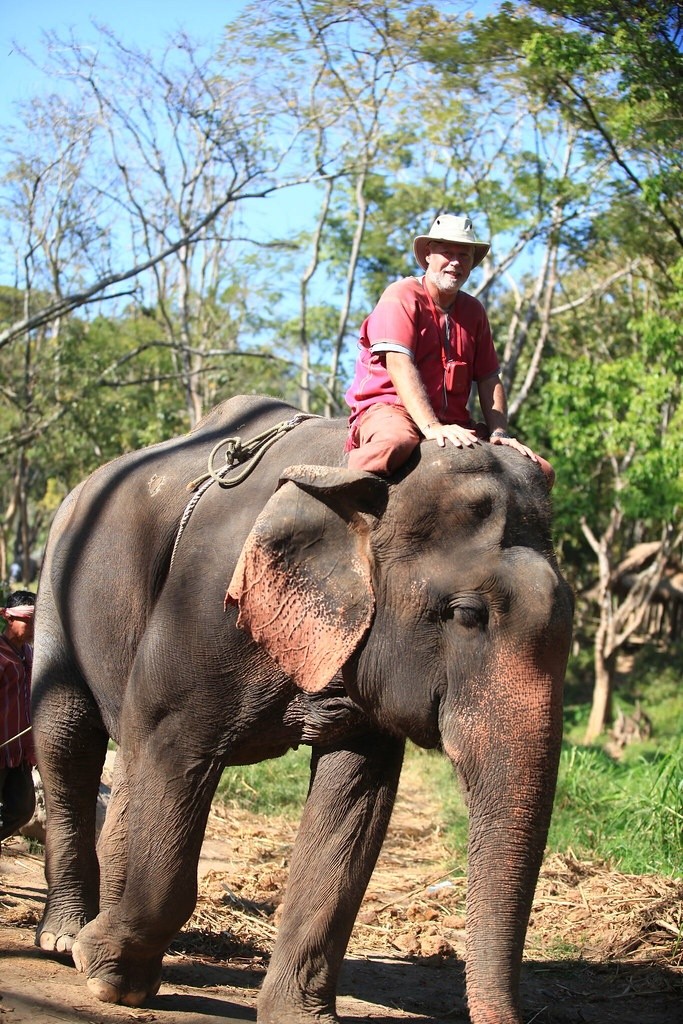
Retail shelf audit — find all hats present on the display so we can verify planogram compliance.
[414,215,490,272]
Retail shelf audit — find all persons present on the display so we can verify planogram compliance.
[344,214,558,492]
[0,590,37,842]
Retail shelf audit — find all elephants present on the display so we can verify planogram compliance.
[30,392,580,1024]
